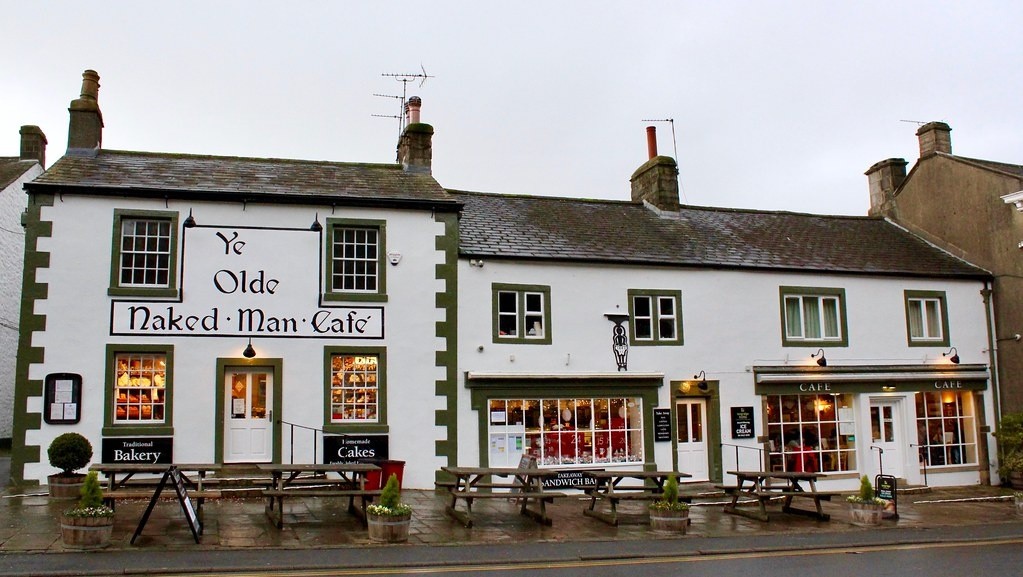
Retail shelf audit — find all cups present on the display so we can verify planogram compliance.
[600,448,605,454]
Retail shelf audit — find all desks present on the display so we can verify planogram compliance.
[88,464,223,535]
[724,471,831,522]
[256,463,382,531]
[440,467,559,529]
[583,471,693,526]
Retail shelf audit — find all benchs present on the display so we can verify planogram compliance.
[574,486,703,498]
[97,481,222,498]
[252,478,383,497]
[434,482,568,498]
[714,485,842,496]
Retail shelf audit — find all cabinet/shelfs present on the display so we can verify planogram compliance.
[333,369,377,410]
[116,371,165,417]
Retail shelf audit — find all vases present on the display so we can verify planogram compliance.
[366,511,412,543]
[649,509,689,535]
[1015,497,1023,516]
[61,515,114,550]
[848,502,883,527]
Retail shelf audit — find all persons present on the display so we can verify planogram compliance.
[917,422,967,465]
[775,426,832,464]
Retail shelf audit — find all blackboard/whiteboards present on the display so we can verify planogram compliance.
[138,467,201,537]
[509,454,543,499]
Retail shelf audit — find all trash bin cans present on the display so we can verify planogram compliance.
[381,461,406,490]
[358,457,380,493]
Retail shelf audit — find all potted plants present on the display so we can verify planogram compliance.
[991,413,1023,491]
[46,432,95,496]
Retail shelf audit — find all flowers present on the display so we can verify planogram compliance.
[648,473,690,512]
[846,474,886,505]
[1013,491,1023,498]
[365,472,412,517]
[61,469,114,518]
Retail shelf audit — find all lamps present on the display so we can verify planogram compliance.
[813,395,831,411]
[183,207,196,228]
[243,336,257,358]
[311,212,323,232]
[811,348,826,367]
[562,408,571,421]
[943,347,960,364]
[694,370,708,390]
[618,405,625,419]
